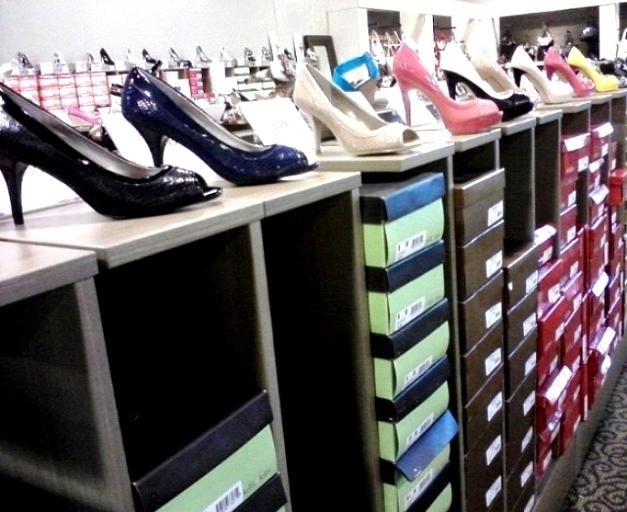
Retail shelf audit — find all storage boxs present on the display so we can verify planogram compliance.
[0,62,627,512]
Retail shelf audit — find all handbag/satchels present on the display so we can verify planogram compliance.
[372,33,404,88]
[523,41,537,59]
[501,37,516,58]
[538,34,553,61]
[581,22,597,40]
[616,28,627,60]
[558,32,578,56]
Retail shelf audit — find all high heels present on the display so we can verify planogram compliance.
[15,47,192,70]
[294,63,420,156]
[196,44,318,67]
[394,42,619,133]
[0,82,222,225]
[122,64,309,185]
